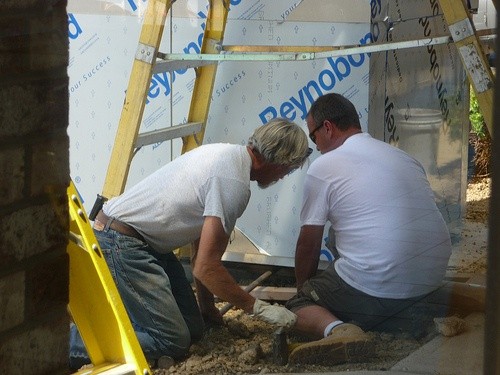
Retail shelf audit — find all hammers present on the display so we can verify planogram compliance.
[272,328,318,367]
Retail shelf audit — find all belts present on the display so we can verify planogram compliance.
[96,210,143,240]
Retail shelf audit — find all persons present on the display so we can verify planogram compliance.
[68,117,311,371]
[280,93,452,367]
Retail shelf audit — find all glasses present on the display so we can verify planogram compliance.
[309,120,328,143]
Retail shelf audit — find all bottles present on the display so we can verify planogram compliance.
[273,330,288,366]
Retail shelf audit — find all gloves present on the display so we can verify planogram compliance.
[254,296,298,335]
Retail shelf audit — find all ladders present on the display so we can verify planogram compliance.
[89,0,497,267]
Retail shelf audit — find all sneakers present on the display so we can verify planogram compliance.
[287,322,371,365]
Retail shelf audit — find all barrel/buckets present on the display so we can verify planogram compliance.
[396,109,444,174]
